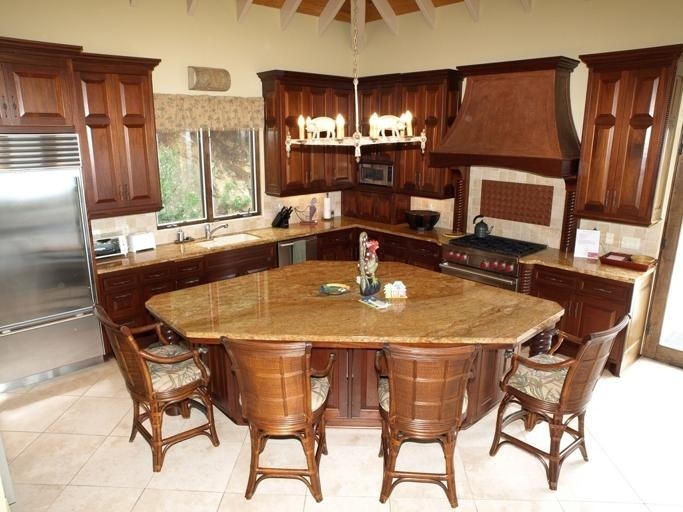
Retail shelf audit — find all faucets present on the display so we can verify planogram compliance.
[203,223,228,240]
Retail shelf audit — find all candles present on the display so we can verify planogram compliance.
[297,114,304,139]
[305,116,312,138]
[404,111,413,136]
[370,112,380,138]
[335,113,345,139]
[400,113,405,136]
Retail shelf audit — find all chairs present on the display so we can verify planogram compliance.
[374,340,483,508]
[92,306,221,471]
[221,335,335,501]
[489,315,632,492]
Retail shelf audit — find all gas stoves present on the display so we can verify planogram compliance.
[447,233,547,278]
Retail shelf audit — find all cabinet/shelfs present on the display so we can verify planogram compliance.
[571,49,682,227]
[256,70,358,192]
[355,69,463,199]
[519,249,658,377]
[97,218,464,363]
[0,35,82,132]
[70,52,163,218]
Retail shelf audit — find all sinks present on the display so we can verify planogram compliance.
[194,232,262,249]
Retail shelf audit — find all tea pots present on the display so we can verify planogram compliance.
[473,215,494,238]
